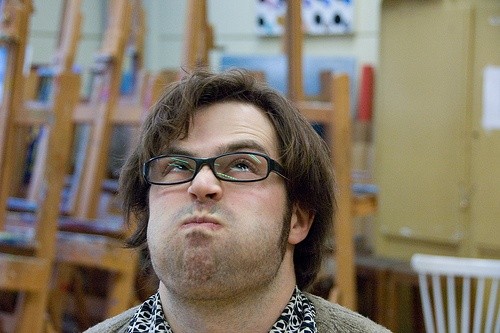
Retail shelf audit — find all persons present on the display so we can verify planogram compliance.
[80,66,393,333]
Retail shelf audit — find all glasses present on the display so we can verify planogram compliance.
[142,153,293,185]
